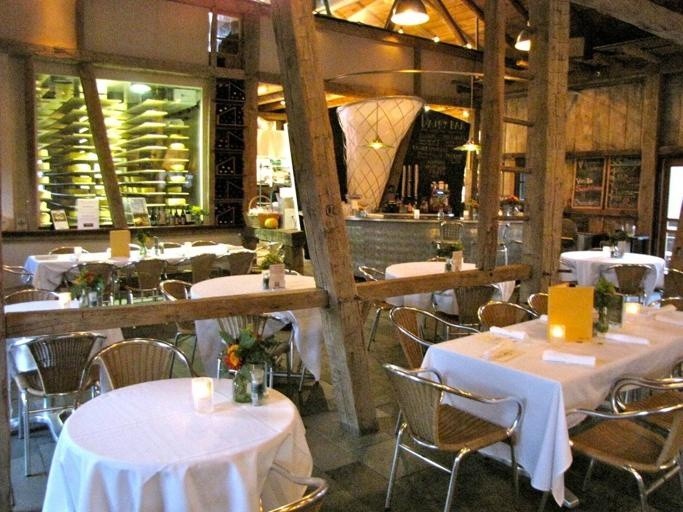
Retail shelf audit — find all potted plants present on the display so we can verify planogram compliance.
[592,275,617,334]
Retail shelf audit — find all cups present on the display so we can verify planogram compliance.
[624,296,642,323]
[191,377,215,415]
[548,324,566,350]
[622,222,637,237]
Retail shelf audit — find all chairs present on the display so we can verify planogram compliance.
[535,360,683,512]
[216,311,316,417]
[561,218,682,310]
[3,287,125,478]
[158,278,197,380]
[261,463,332,512]
[2,266,35,288]
[74,337,195,412]
[433,221,525,264]
[381,363,526,512]
[26,240,261,304]
[358,261,548,366]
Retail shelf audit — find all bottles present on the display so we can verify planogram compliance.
[249,365,265,408]
[137,207,191,258]
[430,180,449,208]
[437,208,444,219]
[444,260,452,273]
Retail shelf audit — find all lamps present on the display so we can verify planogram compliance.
[389,1,430,28]
[355,71,393,151]
[450,74,482,153]
[513,19,532,52]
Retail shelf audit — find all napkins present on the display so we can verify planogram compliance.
[654,315,680,326]
[601,331,649,345]
[541,350,596,365]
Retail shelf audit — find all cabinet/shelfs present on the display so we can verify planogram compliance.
[35,76,195,228]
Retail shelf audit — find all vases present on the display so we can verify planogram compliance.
[507,204,519,216]
[79,288,100,307]
[461,210,473,221]
[436,207,445,220]
[233,364,252,403]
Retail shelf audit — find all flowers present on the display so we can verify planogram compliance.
[223,344,245,371]
[84,270,96,285]
[504,194,520,207]
[431,194,449,210]
[462,197,479,210]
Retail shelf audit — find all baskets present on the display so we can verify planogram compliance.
[242,194,280,228]
